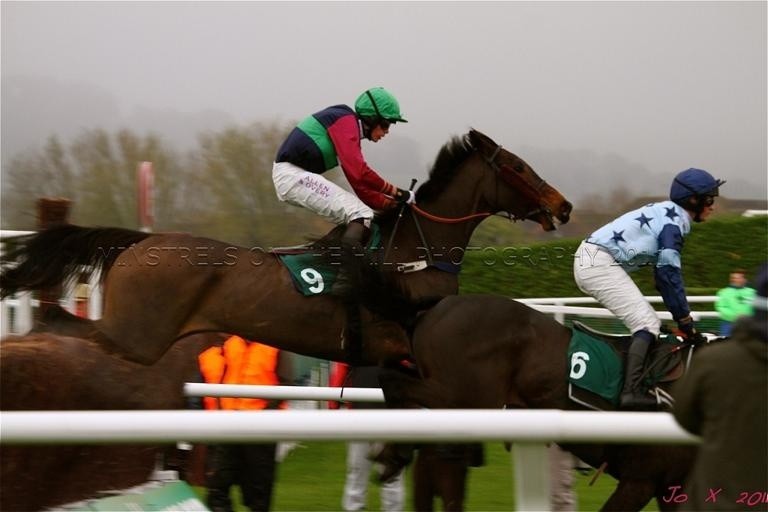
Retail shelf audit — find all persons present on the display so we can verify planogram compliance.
[713,268,758,340]
[328,360,407,511]
[188,335,295,512]
[270,87,416,300]
[573,165,728,411]
[669,266,768,512]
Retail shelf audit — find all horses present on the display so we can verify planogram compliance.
[413,291,698,511]
[0,126,573,511]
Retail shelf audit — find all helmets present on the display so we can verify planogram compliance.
[670,168,721,206]
[353,86,408,128]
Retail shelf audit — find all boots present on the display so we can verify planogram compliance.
[329,222,375,299]
[618,330,669,408]
